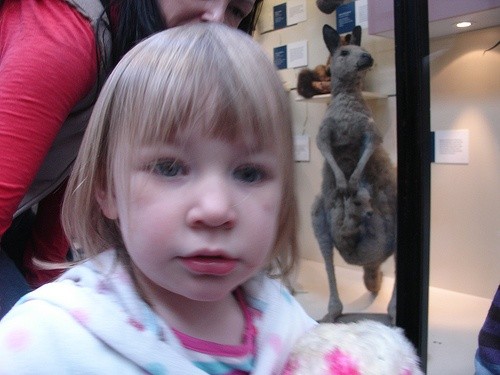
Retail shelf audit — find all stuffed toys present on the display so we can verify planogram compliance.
[282,319,424,375]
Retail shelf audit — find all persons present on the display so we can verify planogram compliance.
[0,22,322,375]
[0,0,264,320]
